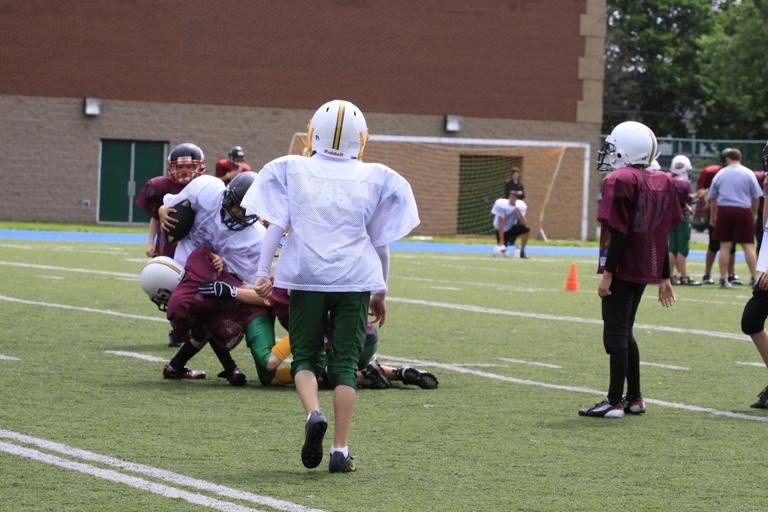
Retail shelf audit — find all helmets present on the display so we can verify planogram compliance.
[219,169,258,231]
[228,146,244,165]
[596,120,657,172]
[309,99,368,160]
[140,256,185,311]
[669,153,693,176]
[165,143,206,183]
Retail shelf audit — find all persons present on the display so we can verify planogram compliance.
[240,99,421,472]
[491,190,530,258]
[742,218,767,409]
[579,120,681,419]
[504,167,526,200]
[646,148,768,289]
[137,142,439,390]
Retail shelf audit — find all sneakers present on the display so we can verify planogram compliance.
[751,388,767,408]
[226,367,246,386]
[328,450,356,473]
[520,250,527,258]
[361,358,391,389]
[669,273,742,288]
[163,363,207,380]
[301,412,327,468]
[397,366,437,389]
[578,395,645,419]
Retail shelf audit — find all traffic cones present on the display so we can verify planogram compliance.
[565,262,579,291]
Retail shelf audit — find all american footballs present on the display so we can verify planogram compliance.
[164,200,196,244]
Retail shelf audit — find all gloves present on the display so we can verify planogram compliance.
[197,280,237,302]
